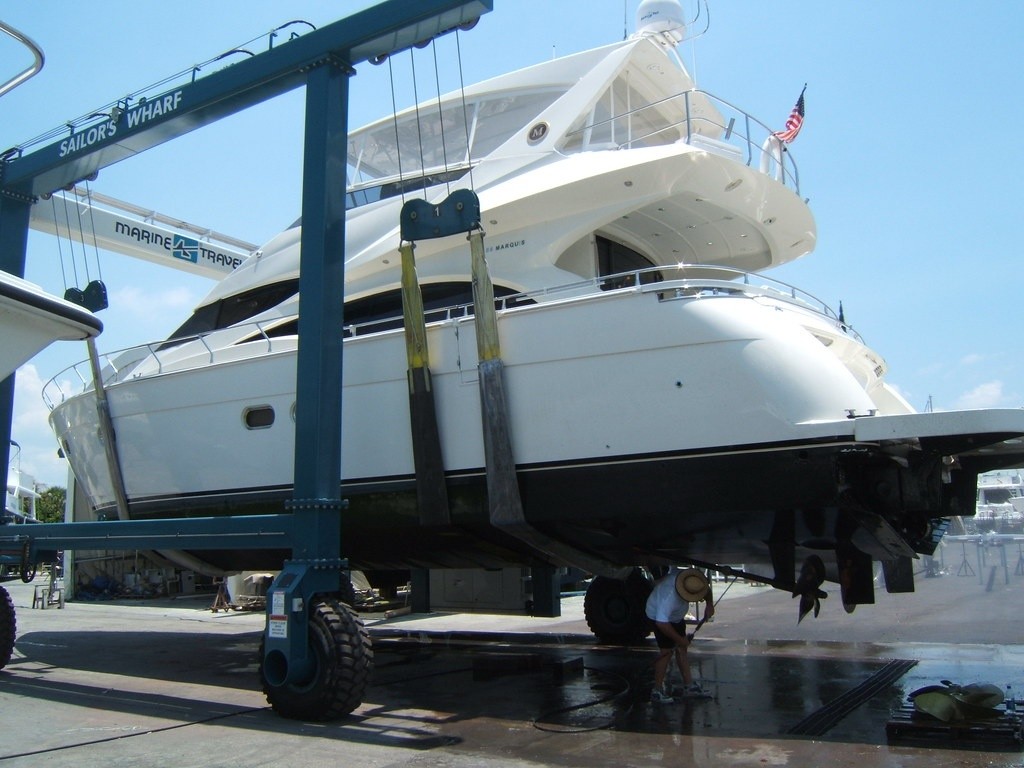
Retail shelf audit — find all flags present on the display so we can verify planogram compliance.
[770,86,805,144]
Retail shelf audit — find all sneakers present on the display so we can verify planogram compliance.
[649,687,673,703]
[683,681,712,698]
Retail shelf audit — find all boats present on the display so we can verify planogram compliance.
[39,3,1022,647]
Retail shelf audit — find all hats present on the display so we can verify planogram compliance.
[675,569,710,603]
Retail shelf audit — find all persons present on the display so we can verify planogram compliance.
[643,569,715,705]
